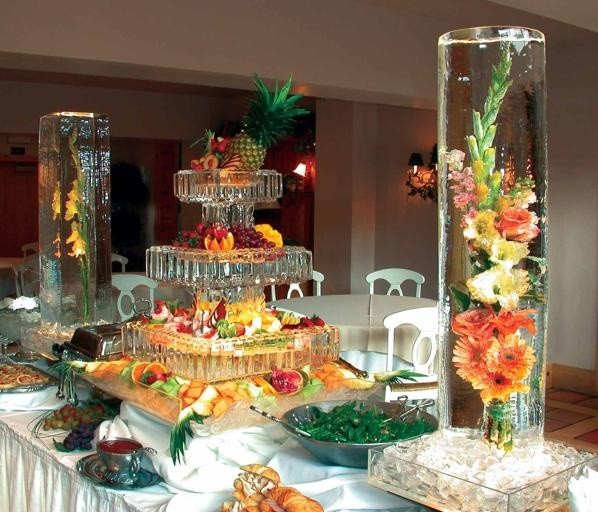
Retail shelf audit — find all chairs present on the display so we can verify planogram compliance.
[14,241,159,325]
[269,266,441,406]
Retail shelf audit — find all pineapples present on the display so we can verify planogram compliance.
[218,71,314,172]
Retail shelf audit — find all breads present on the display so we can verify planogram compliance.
[222,464,324,512]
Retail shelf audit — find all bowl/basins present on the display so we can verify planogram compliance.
[280,399,439,468]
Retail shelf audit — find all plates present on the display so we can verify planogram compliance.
[76,453,163,490]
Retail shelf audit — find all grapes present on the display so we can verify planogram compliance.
[231,224,284,260]
[61,421,100,450]
[45,404,104,433]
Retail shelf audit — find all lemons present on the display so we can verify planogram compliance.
[132,362,147,385]
[144,362,166,374]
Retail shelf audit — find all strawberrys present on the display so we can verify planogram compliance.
[172,223,203,249]
[312,314,325,326]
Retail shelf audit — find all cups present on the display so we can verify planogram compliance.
[96,438,144,485]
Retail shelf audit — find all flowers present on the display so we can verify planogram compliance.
[445,59,548,422]
[42,123,99,327]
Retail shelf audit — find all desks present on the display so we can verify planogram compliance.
[0,334,459,512]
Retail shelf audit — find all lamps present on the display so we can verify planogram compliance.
[292,162,307,179]
[408,152,424,177]
